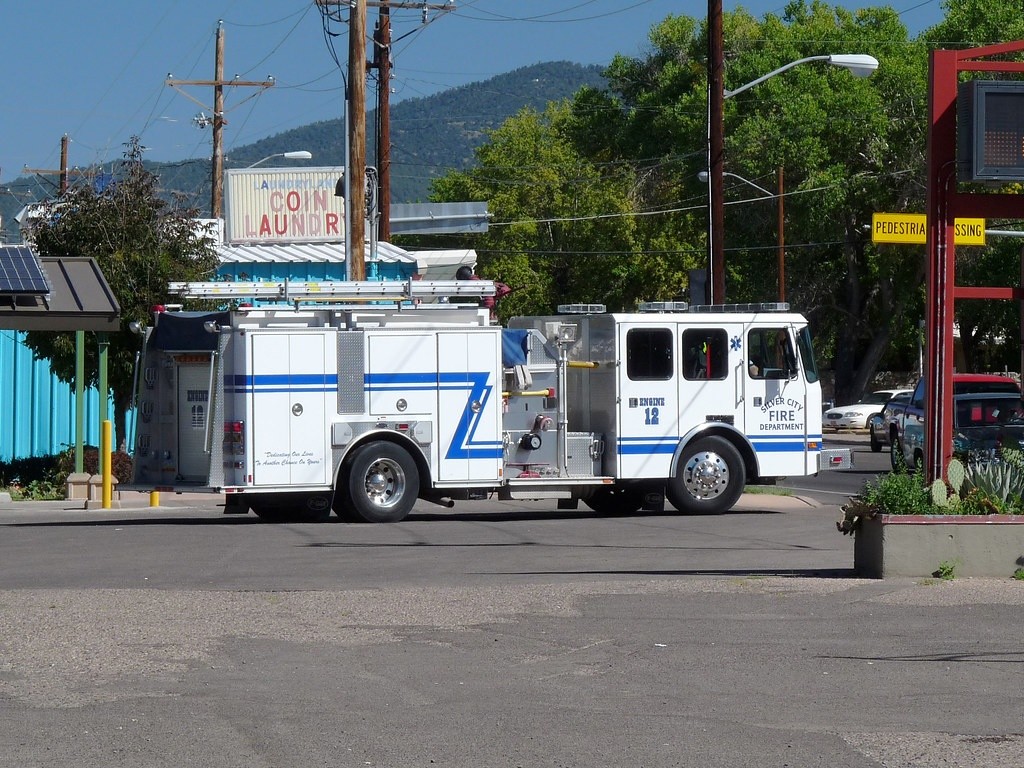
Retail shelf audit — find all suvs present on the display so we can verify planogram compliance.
[885,374,1024,473]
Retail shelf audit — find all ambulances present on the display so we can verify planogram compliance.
[114,276,854,525]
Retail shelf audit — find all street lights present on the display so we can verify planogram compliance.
[212,150,313,219]
[709,54,879,306]
[699,171,785,302]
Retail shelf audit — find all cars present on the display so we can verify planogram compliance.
[823,390,916,429]
[952,393,1024,469]
[870,397,912,453]
